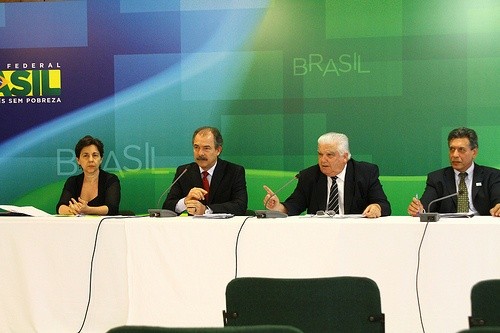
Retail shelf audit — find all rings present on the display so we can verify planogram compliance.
[194,208,197,212]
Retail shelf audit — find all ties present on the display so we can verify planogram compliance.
[457,172,470,213]
[328,177,339,214]
[201,172,209,202]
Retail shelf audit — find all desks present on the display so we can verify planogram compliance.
[0,214,500,333]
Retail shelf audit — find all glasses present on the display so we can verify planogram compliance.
[311,211,336,218]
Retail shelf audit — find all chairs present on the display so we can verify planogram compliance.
[467,278,500,330]
[221,276,385,333]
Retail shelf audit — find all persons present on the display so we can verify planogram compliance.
[162,126,248,216]
[263,132,391,218]
[408,127,500,217]
[56,135,121,216]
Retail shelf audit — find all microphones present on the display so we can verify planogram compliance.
[420,190,464,222]
[256,174,301,218]
[148,164,192,217]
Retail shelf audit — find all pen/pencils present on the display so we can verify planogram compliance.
[416,194,420,217]
